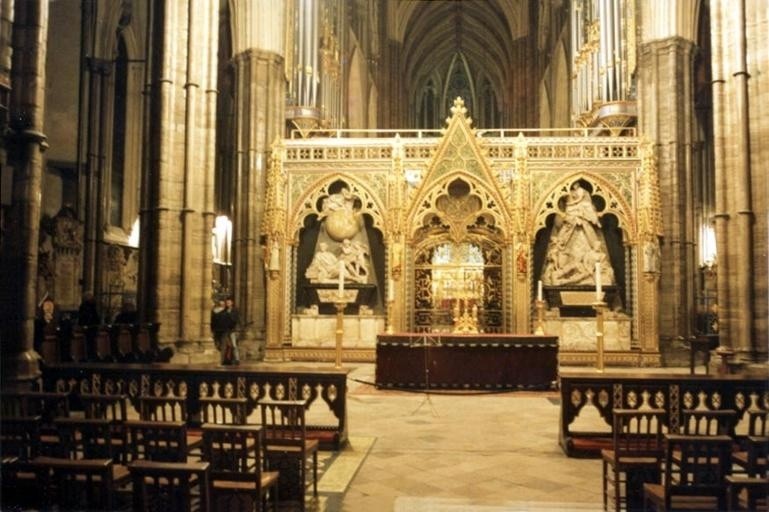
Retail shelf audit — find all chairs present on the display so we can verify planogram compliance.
[749,408,767,432]
[598,407,664,511]
[732,436,768,475]
[667,407,738,466]
[139,396,200,452]
[20,392,80,444]
[202,425,282,512]
[726,477,769,511]
[0,413,54,480]
[25,458,117,512]
[643,433,731,512]
[67,419,130,485]
[256,399,319,511]
[87,397,142,448]
[196,396,256,466]
[2,392,24,414]
[127,458,210,511]
[141,421,198,486]
[37,421,72,454]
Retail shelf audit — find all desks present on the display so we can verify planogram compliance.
[374,332,560,390]
[687,336,718,375]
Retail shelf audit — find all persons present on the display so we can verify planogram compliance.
[78,289,101,362]
[224,297,241,363]
[54,311,73,364]
[211,300,235,365]
[35,308,47,357]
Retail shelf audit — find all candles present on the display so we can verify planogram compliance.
[537,278,543,303]
[337,259,345,297]
[595,260,602,301]
